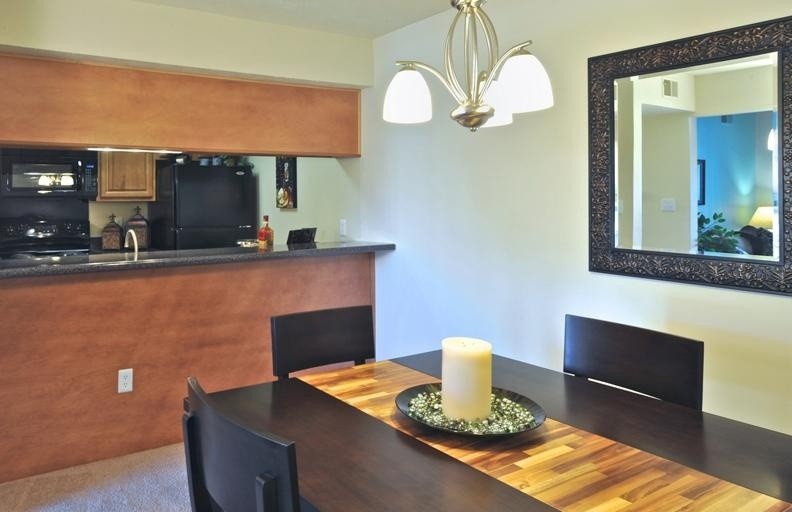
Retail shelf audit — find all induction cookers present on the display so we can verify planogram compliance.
[0,216,91,256]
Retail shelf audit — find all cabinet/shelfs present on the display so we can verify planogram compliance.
[93,150,156,205]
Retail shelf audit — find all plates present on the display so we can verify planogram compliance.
[394,382,547,438]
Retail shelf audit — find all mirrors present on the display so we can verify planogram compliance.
[586,15,792,298]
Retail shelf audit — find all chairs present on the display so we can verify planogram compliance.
[560,312,706,411]
[269,301,377,380]
[180,374,301,512]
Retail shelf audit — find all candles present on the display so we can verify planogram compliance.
[440,335,494,425]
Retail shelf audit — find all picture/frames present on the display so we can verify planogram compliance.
[697,159,706,205]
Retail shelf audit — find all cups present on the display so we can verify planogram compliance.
[198,155,222,166]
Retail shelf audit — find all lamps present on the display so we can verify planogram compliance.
[381,0,554,132]
[748,206,774,229]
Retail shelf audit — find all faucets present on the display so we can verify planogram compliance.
[124,228,138,261]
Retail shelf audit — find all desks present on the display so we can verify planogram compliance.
[181,347,792,512]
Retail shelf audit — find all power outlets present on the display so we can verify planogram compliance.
[117,368,133,394]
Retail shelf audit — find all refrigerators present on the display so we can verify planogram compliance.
[146,159,258,251]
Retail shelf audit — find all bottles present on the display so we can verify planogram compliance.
[258,215,274,248]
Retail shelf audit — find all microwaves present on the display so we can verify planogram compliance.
[0,149,99,202]
